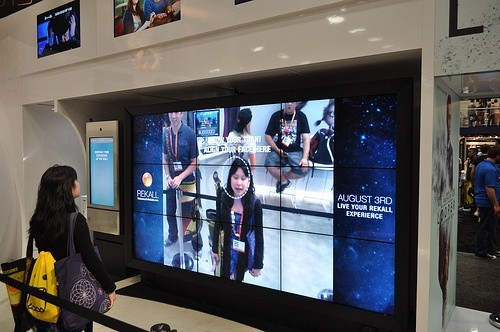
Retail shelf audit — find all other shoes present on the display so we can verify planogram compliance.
[164,238,173,246]
[276,179,291,193]
[193,250,201,260]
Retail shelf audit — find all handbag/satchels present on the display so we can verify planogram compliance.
[246,197,258,270]
[0,255,35,332]
[54,212,112,331]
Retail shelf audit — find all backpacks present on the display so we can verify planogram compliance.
[309,127,334,164]
[26,229,61,323]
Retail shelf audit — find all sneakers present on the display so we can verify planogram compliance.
[475,253,496,259]
[492,251,500,256]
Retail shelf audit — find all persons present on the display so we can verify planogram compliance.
[211,158,264,282]
[436,94,454,329]
[25,163,118,332]
[263,101,311,193]
[225,108,256,172]
[459,138,500,260]
[162,111,205,253]
[142,0,175,19]
[41,9,81,55]
[468,99,500,127]
[123,0,157,35]
